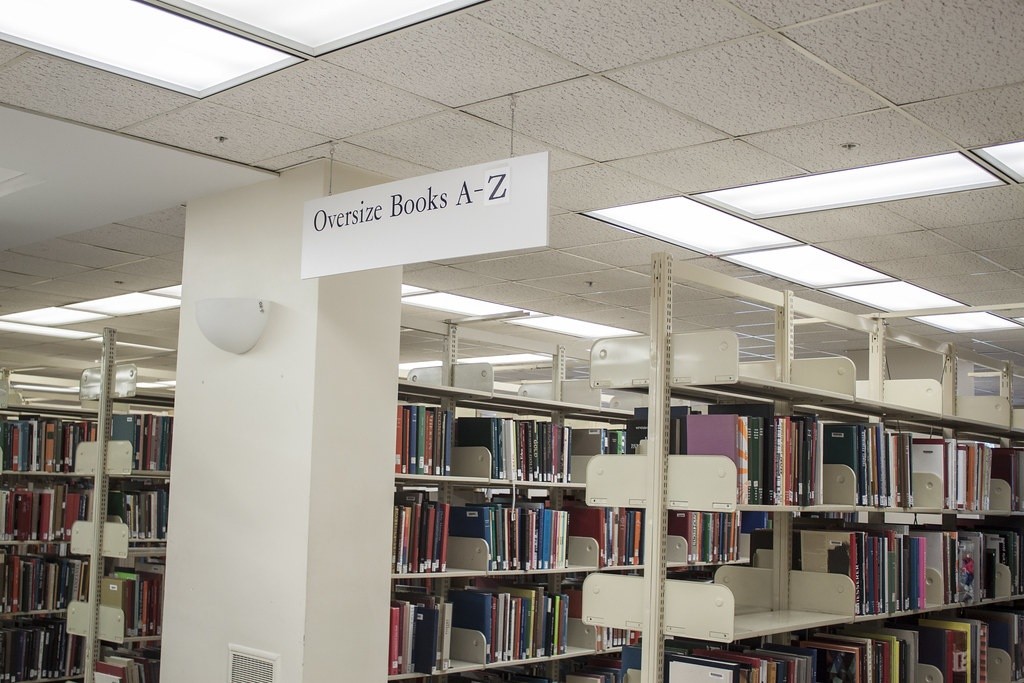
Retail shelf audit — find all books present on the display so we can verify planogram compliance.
[626,399,1024,683]
[387,404,640,683]
[0,414,174,683]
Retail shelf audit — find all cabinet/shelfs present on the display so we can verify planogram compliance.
[65,366,175,683]
[583,340,1024,683]
[390,380,643,683]
[0,401,116,683]
